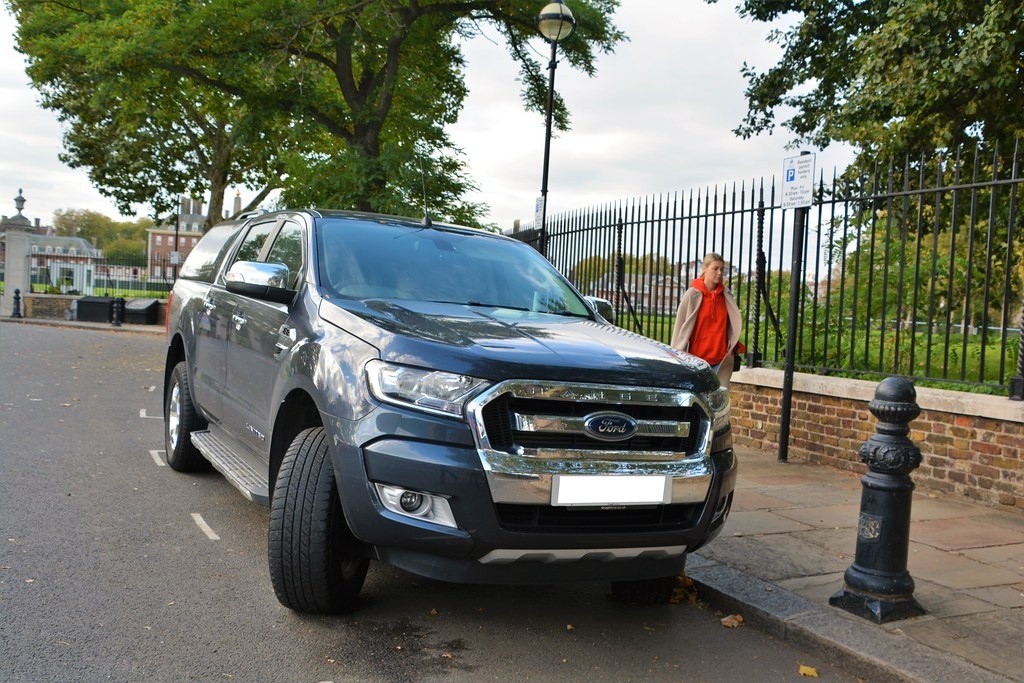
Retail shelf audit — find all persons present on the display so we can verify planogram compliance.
[671,253,747,395]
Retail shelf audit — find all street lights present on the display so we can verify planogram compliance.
[533,2,579,254]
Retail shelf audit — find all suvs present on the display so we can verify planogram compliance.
[164,210,738,625]
